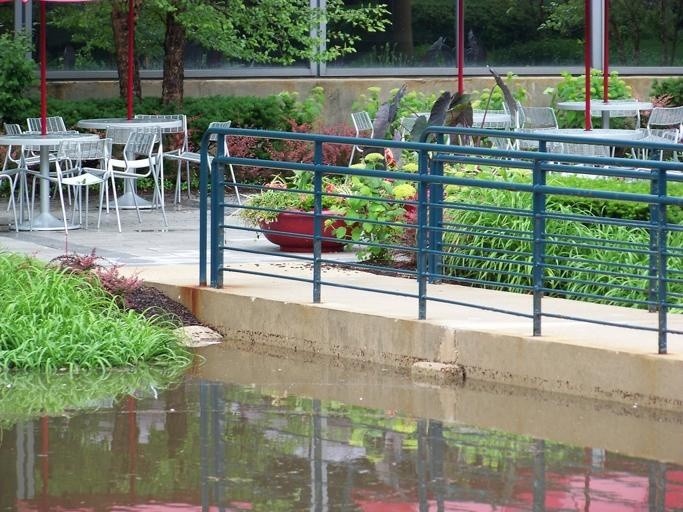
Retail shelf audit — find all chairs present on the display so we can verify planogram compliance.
[0,114,240,237]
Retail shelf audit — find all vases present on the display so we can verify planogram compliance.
[255,208,360,252]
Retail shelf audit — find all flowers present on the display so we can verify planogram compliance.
[227,161,367,239]
